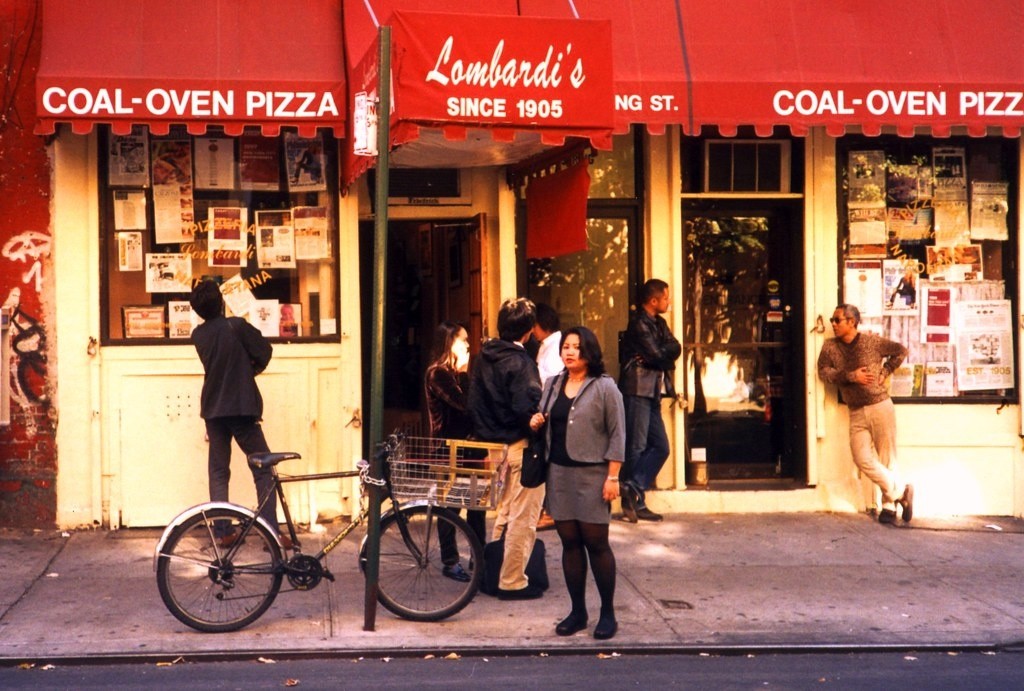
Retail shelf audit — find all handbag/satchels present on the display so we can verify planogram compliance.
[476,522,550,597]
[518,375,560,489]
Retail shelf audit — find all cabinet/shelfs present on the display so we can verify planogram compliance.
[386,265,420,411]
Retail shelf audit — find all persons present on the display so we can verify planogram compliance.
[529,326,624,641]
[424,298,565,600]
[818,304,914,524]
[189,280,302,548]
[618,279,680,523]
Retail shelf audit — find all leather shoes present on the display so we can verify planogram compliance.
[442,563,471,581]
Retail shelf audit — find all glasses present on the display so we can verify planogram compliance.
[831,317,854,323]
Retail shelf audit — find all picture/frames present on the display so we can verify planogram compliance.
[392,239,406,286]
[447,229,464,290]
[416,222,434,277]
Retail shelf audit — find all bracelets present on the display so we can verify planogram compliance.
[607,476,618,481]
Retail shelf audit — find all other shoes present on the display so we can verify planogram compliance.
[496,586,544,599]
[620,484,639,523]
[556,612,589,638]
[901,485,915,523]
[594,623,618,639]
[262,532,301,552]
[878,508,895,525]
[636,509,665,522]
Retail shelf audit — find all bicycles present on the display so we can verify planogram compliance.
[151,424,511,633]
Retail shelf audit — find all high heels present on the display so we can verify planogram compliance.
[220,532,245,547]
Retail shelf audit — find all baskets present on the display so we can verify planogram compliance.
[388,436,510,511]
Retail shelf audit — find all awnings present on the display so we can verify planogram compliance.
[31,1,1024,140]
